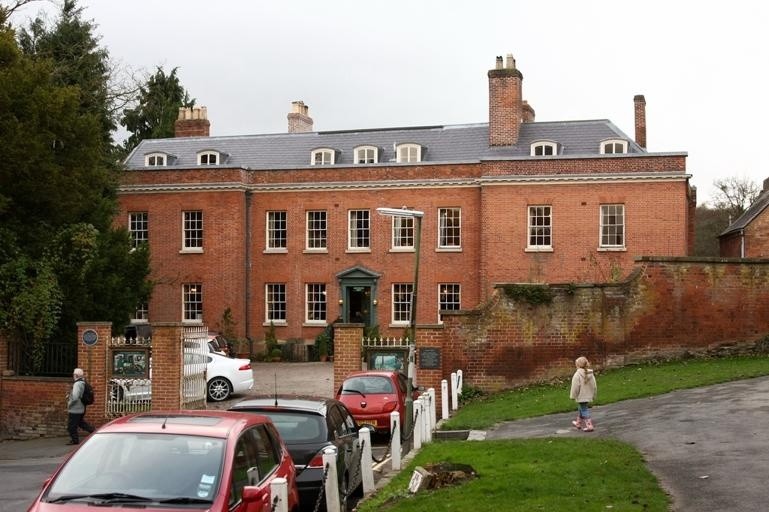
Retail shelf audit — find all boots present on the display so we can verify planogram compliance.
[572,417,593,431]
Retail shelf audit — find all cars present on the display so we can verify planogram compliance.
[114,339,227,371]
[26,409,300,511]
[184,330,235,357]
[108,347,255,412]
[183,335,229,357]
[223,371,377,512]
[334,368,425,441]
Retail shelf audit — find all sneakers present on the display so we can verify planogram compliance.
[64,441,79,444]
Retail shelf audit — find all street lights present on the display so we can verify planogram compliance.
[375,205,424,459]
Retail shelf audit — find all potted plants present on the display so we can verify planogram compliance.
[235,337,249,359]
[317,333,329,362]
[271,348,283,361]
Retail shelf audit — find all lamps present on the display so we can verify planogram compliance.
[372,299,378,306]
[338,298,344,306]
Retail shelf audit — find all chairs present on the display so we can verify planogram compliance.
[351,380,364,392]
[384,382,393,392]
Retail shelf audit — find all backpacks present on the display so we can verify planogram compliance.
[74,378,93,405]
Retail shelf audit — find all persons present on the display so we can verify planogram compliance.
[65,368,97,445]
[568,356,598,431]
